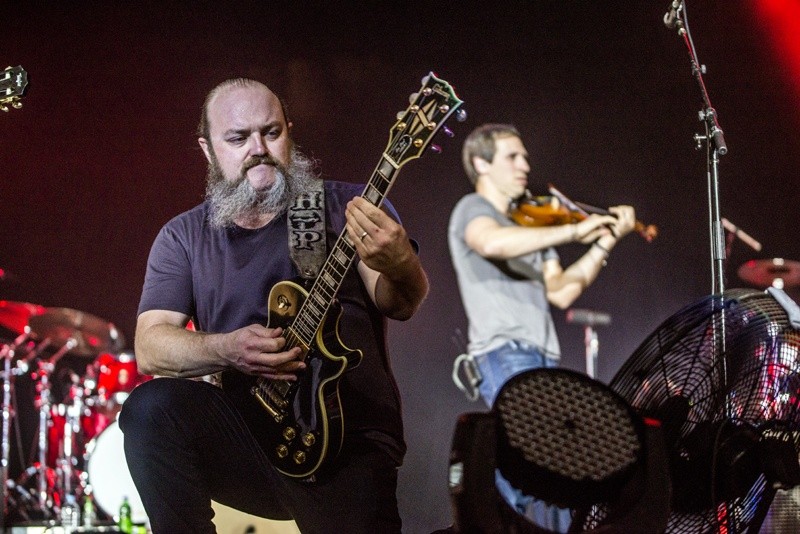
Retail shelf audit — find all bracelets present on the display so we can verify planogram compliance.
[592,241,611,257]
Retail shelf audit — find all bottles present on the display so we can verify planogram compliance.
[60,494,81,527]
[118,497,133,534]
[83,497,94,527]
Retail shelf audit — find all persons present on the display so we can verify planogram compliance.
[118,78,430,534]
[447,123,636,532]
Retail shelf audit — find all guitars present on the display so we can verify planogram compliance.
[221,71,468,479]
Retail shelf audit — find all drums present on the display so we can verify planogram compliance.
[80,405,115,456]
[97,353,159,406]
[36,404,75,470]
[79,418,150,525]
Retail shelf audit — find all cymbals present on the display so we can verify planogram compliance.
[30,308,125,358]
[0,300,45,334]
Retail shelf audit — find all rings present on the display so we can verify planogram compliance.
[360,231,367,241]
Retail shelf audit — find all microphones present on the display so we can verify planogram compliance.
[663,0,683,30]
[566,308,612,327]
[721,220,762,251]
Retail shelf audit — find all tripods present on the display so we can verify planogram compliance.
[0,327,92,526]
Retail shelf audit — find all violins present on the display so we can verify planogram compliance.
[506,192,659,241]
